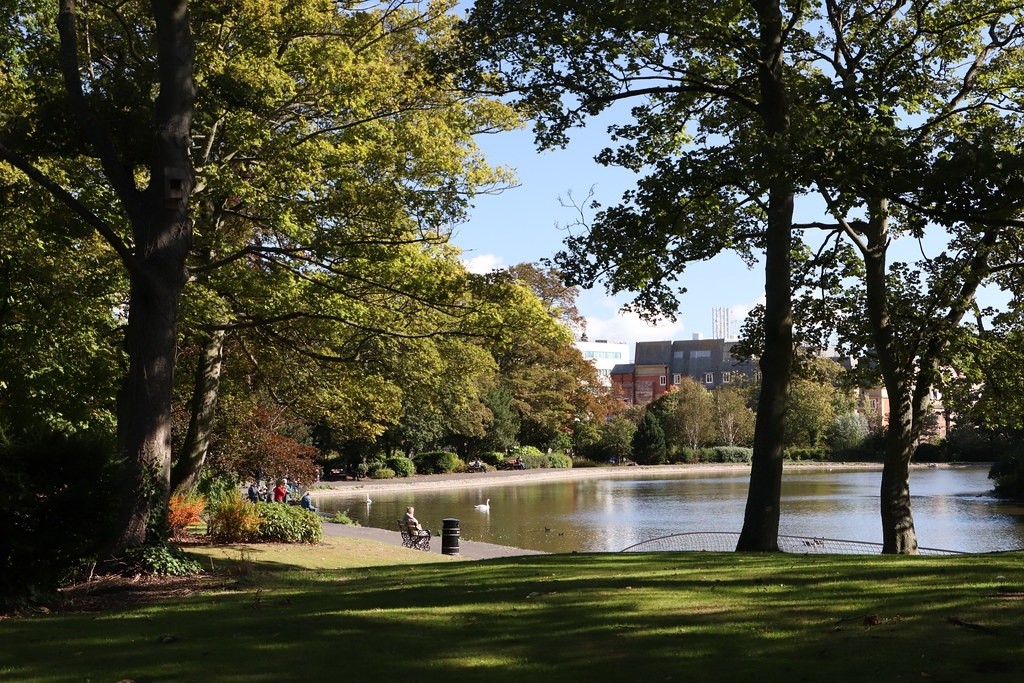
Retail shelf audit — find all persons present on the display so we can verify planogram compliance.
[517,456,526,470]
[404,507,428,549]
[609,455,626,464]
[248,476,318,513]
[469,456,489,474]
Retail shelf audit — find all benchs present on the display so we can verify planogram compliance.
[507,464,521,470]
[397,519,431,551]
[468,466,482,473]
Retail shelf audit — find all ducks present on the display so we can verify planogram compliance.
[801,536,826,547]
[364,494,373,505]
[473,498,491,510]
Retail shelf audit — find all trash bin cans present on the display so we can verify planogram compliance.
[441,518,461,556]
